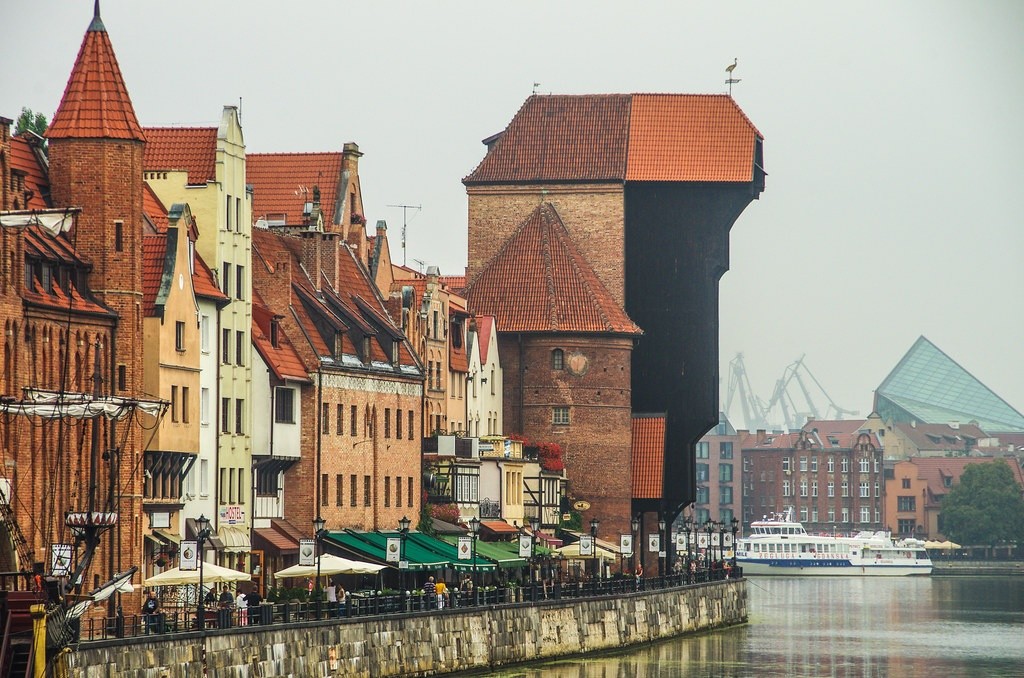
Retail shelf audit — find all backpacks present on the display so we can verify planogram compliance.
[462,580,469,591]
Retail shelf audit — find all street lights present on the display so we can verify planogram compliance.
[398,514,412,613]
[589,514,600,595]
[702,517,714,571]
[312,515,327,620]
[193,514,211,629]
[691,519,701,584]
[528,515,542,601]
[468,516,482,606]
[685,515,693,585]
[717,518,727,562]
[630,516,638,593]
[731,516,740,579]
[102,446,127,637]
[659,517,669,590]
[69,525,84,644]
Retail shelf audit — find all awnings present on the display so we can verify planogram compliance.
[320,533,557,572]
[146,529,183,555]
[220,526,252,552]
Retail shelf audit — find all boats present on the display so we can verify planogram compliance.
[733,505,935,577]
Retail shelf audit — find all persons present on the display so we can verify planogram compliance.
[458,574,473,595]
[323,577,337,617]
[220,585,234,602]
[636,564,642,577]
[673,558,733,580]
[336,583,345,615]
[557,567,566,580]
[236,589,247,626]
[423,576,436,608]
[141,592,161,634]
[205,588,218,601]
[435,578,448,608]
[243,585,263,624]
[307,576,314,594]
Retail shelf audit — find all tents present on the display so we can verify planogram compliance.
[552,540,616,575]
[273,552,388,597]
[144,559,253,630]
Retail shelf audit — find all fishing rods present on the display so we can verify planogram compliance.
[744,577,780,600]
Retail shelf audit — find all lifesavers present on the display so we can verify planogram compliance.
[819,532,829,537]
[810,548,816,553]
[836,533,843,537]
[906,552,912,558]
[876,554,882,559]
